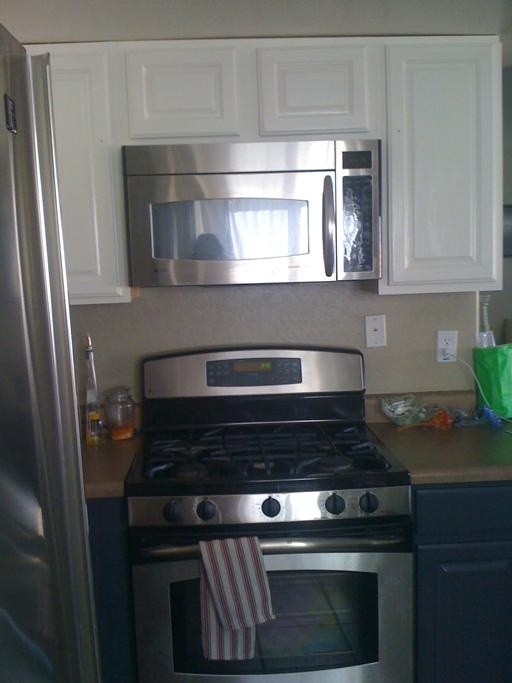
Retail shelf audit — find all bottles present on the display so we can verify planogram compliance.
[105,384,134,441]
[80,336,102,446]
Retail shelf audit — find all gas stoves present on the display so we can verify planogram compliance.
[124,418,413,532]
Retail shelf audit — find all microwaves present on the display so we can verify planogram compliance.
[120,137,386,287]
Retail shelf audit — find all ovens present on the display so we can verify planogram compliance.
[129,526,416,682]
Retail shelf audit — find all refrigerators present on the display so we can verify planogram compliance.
[1,21,103,682]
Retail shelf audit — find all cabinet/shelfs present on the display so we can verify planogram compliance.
[383,36,503,295]
[406,477,512,681]
[23,40,131,306]
[85,491,142,681]
[125,37,383,145]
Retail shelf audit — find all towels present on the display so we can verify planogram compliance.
[198,533,276,664]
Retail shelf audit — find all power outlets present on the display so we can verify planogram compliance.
[437,330,458,362]
